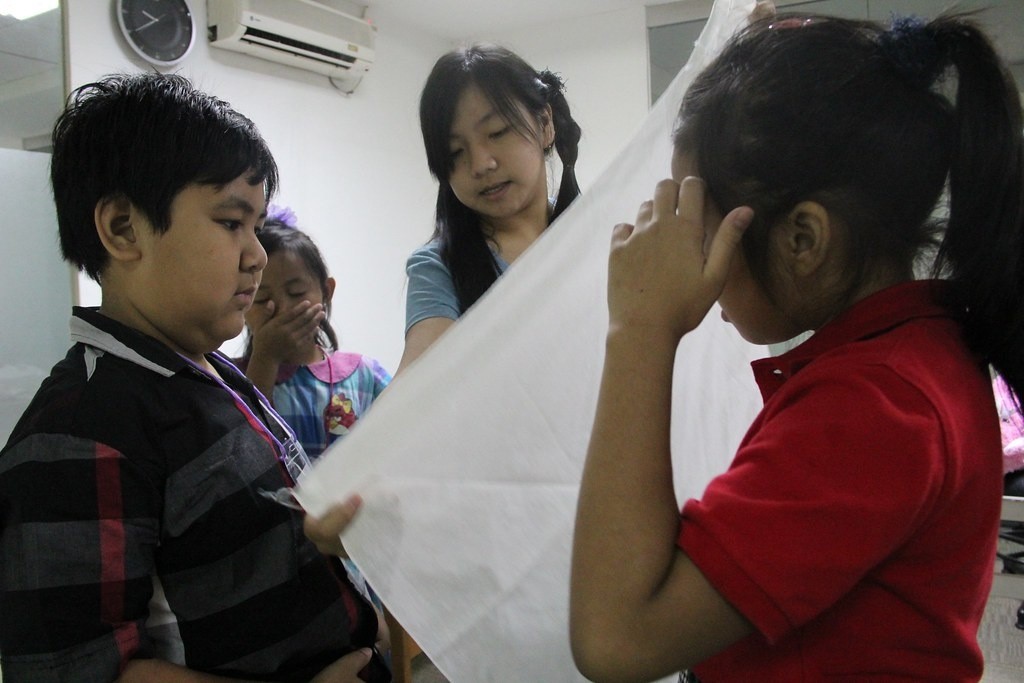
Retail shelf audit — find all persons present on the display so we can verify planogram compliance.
[231,205,391,664]
[0,73,394,683]
[570,0,1024,683]
[391,44,581,380]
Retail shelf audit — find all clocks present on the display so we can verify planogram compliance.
[115,0,196,66]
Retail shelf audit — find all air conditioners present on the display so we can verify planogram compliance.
[207,0,375,81]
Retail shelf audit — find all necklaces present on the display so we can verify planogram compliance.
[312,347,333,448]
